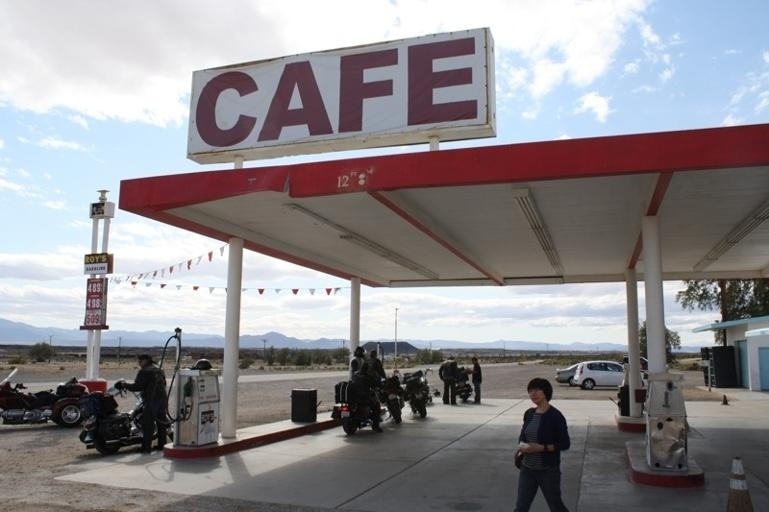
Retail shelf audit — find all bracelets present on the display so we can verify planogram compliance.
[544,445,548,452]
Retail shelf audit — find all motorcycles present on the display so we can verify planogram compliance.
[454,365,472,402]
[0,379,89,428]
[80,391,173,455]
[331,367,433,436]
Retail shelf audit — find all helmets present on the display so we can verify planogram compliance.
[196,359,213,369]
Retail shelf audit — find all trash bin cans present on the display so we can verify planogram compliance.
[292,388,317,422]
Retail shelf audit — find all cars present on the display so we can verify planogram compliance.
[555,360,648,390]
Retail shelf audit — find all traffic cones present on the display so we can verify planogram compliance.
[726,456,755,511]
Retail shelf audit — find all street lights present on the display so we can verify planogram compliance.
[395,308,398,360]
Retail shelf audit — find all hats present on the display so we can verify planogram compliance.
[138,354,157,363]
[354,370,370,377]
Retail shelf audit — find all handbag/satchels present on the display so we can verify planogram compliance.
[515,450,523,469]
[334,381,353,403]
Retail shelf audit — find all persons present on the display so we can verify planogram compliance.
[121,353,168,454]
[351,347,386,384]
[513,378,570,512]
[619,357,629,387]
[468,357,481,404]
[351,362,383,433]
[439,355,457,404]
